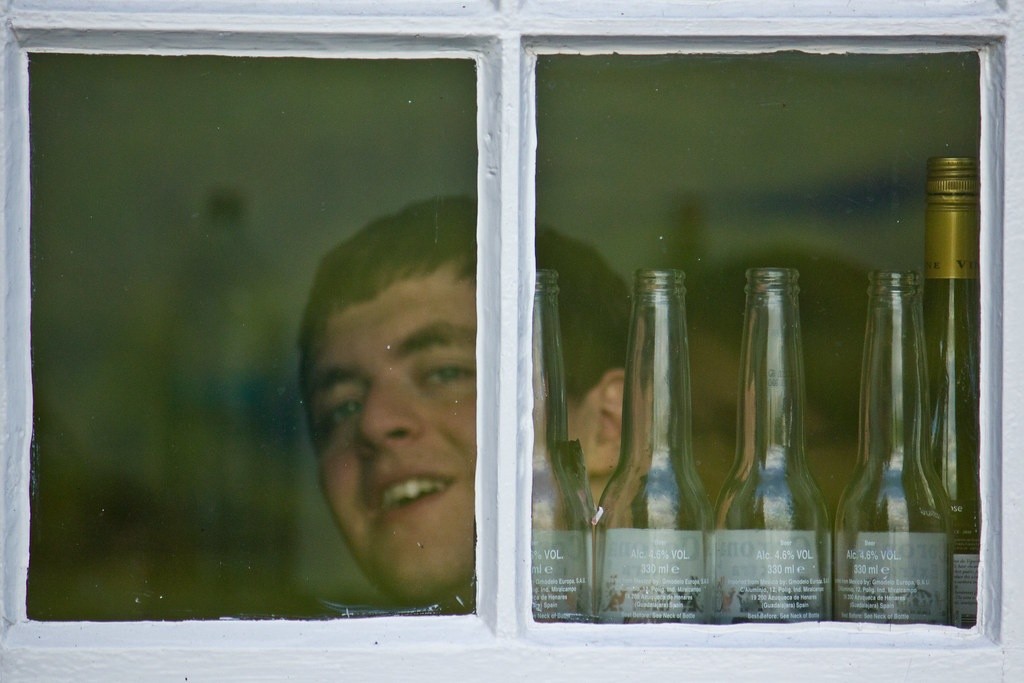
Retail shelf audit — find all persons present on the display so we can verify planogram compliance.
[298,195,654,612]
[670,254,934,524]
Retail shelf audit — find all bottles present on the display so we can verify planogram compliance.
[832,271,948,623]
[715,266,829,622]
[158,194,293,619]
[532,270,596,622]
[923,157,980,626]
[594,269,713,623]
